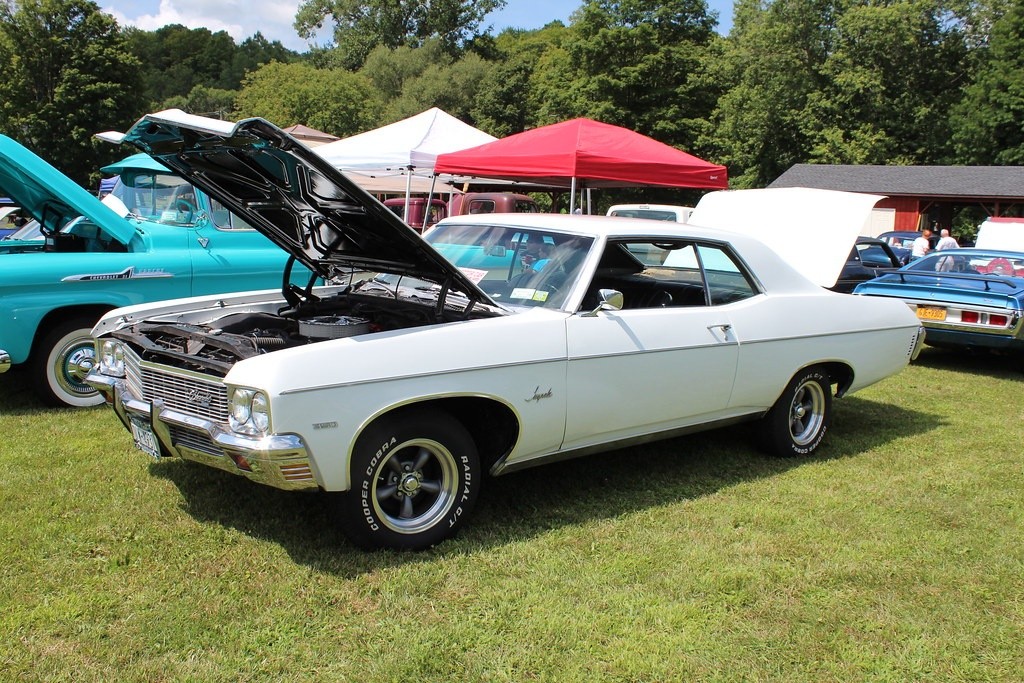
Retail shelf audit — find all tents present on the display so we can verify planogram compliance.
[98,175,187,203]
[310,103,572,224]
[421,117,728,240]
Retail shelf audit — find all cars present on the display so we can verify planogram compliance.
[832,237,903,293]
[87,109,928,554]
[862,231,943,266]
[853,217,1024,357]
[0,135,712,410]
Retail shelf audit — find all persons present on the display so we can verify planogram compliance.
[935,229,965,272]
[560,206,567,214]
[520,233,555,273]
[574,205,582,214]
[911,229,932,262]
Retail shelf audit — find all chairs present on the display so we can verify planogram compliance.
[504,273,534,306]
[525,272,569,306]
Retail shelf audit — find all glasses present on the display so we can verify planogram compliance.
[526,241,533,244]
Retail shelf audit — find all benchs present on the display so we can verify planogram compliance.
[582,274,732,312]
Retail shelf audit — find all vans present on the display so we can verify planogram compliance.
[597,205,696,270]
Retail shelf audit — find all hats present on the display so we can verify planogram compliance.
[528,232,544,243]
